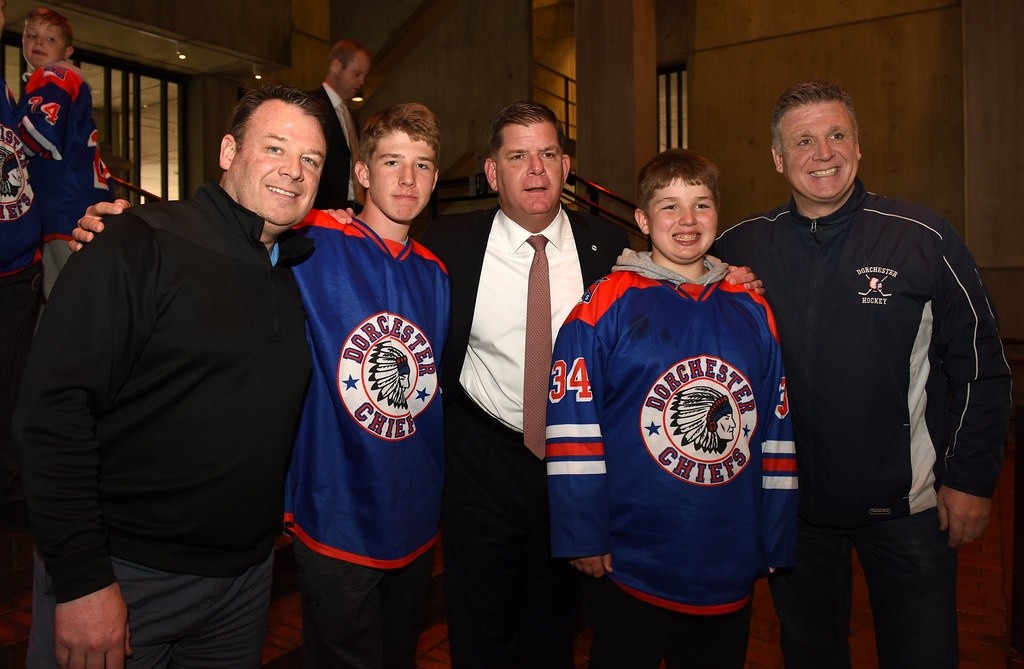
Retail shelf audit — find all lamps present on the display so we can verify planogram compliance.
[351,91,363,101]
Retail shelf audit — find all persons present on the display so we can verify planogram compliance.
[12,82,327,669]
[709,82,1012,669]
[323,100,765,669]
[545,148,798,669]
[307,43,371,216]
[69,103,451,669]
[0,0,113,527]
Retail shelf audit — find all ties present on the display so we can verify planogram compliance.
[340,102,366,206]
[523,235,553,460]
